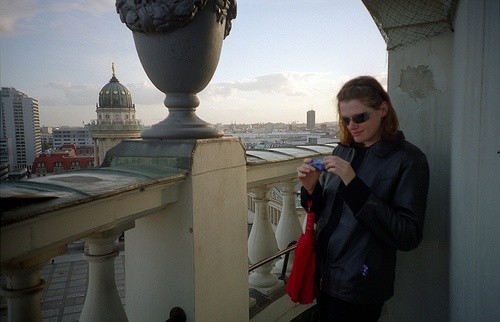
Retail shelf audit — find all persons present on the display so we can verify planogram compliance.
[296,76,430,321]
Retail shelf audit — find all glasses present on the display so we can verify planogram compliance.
[340,107,378,125]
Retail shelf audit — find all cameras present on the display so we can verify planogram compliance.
[309,156,330,172]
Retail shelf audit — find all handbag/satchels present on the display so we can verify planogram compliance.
[284,200,317,305]
